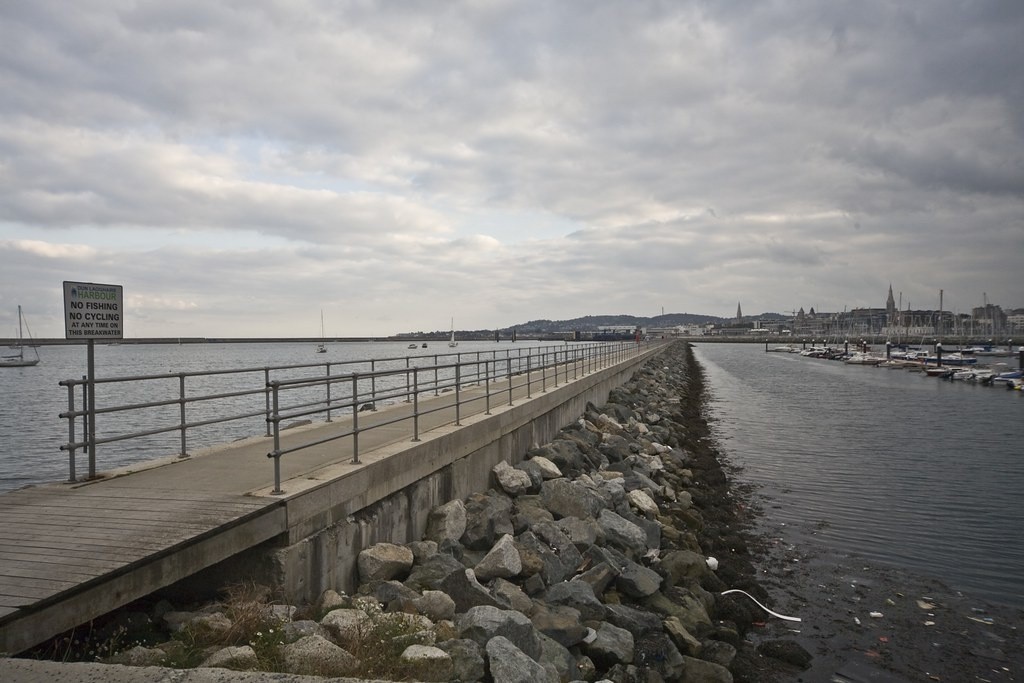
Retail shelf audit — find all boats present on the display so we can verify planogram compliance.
[408,343,418,349]
[421,342,428,349]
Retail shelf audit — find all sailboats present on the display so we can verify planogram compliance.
[773,288,1024,393]
[0,304,41,368]
[447,317,459,347]
[314,309,327,353]
